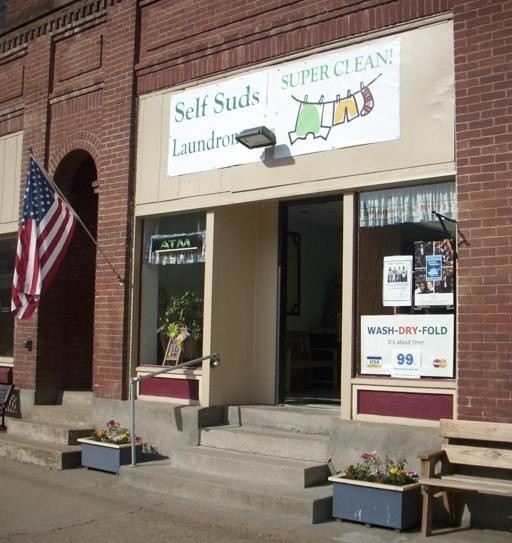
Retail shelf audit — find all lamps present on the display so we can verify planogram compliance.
[236,124,277,150]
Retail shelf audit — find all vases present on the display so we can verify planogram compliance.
[327,472,419,533]
[77,435,144,476]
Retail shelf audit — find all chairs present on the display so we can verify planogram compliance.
[282,328,339,395]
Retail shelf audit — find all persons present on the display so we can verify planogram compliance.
[387,264,408,281]
[415,239,454,293]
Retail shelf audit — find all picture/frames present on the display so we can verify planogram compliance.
[285,229,301,316]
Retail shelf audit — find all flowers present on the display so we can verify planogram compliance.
[342,449,418,488]
[92,419,143,444]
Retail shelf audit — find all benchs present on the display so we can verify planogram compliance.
[415,417,512,537]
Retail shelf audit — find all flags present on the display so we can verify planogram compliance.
[10,156,80,321]
